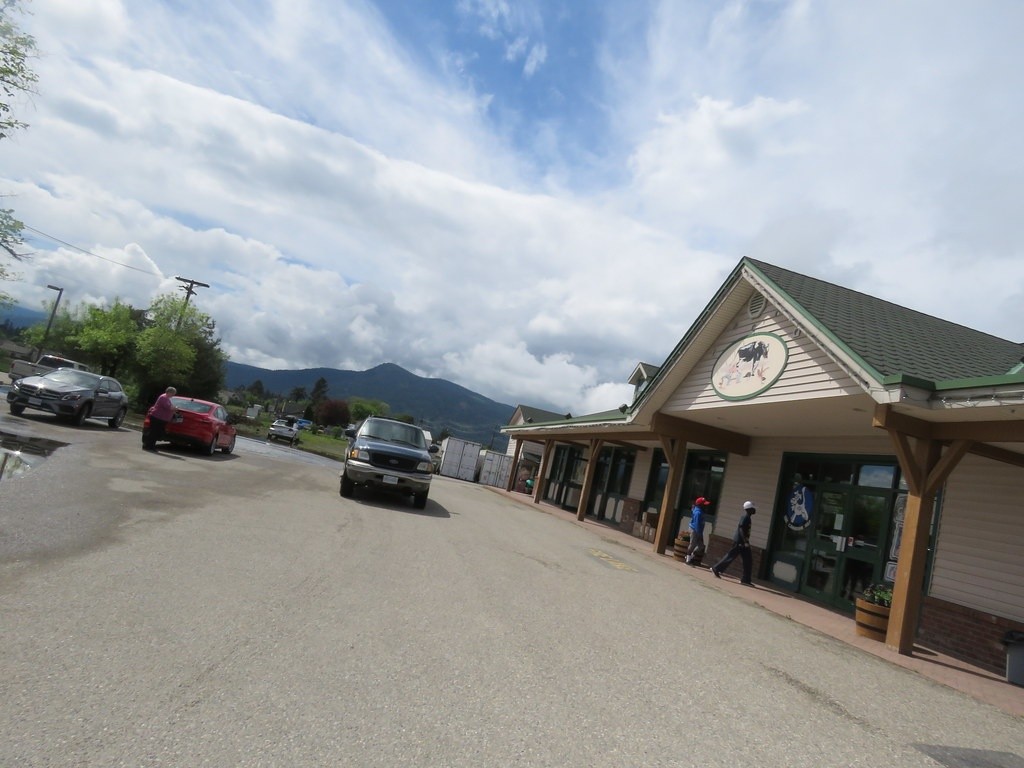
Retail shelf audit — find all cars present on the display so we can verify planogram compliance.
[141,396,237,456]
[267,419,302,448]
[6,368,130,428]
[298,419,314,431]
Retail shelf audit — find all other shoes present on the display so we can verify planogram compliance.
[685,555,694,567]
[739,580,756,588]
[710,566,722,579]
[142,447,159,453]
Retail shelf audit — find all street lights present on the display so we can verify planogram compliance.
[33,284,64,363]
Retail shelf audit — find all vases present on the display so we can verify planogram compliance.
[674,539,706,565]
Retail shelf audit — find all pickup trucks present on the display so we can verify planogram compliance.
[8,354,92,386]
[338,414,441,509]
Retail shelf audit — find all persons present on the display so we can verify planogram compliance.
[684,497,710,565]
[142,386,176,450]
[710,501,757,587]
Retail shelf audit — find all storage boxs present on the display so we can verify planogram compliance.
[620,498,660,543]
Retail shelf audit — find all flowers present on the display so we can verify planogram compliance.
[679,530,691,541]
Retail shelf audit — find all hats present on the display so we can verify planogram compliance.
[743,501,757,510]
[696,497,712,506]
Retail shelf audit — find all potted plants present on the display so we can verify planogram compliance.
[855,584,892,642]
